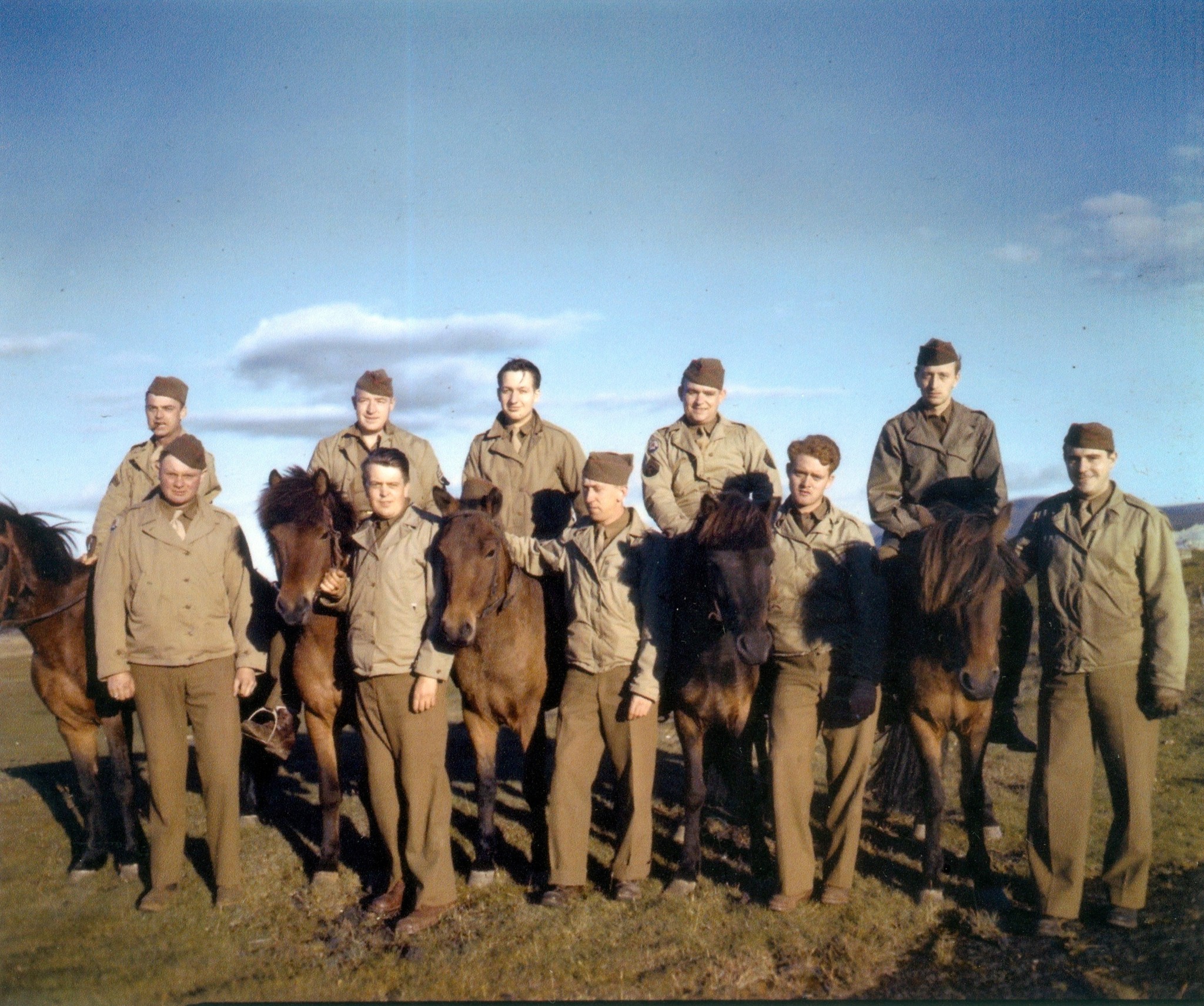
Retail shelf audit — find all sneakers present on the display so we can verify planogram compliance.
[1105,903,1137,928]
[396,899,458,934]
[139,888,176,909]
[987,711,1037,752]
[613,880,642,901]
[214,884,239,905]
[768,888,813,912]
[1037,912,1062,937]
[819,884,849,904]
[369,882,406,913]
[542,885,584,907]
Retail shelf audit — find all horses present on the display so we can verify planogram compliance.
[432,485,584,893]
[643,488,775,896]
[259,466,360,882]
[869,513,1029,916]
[0,495,303,881]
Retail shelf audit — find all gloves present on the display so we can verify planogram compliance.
[1135,683,1183,720]
[845,679,878,725]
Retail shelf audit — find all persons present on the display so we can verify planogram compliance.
[320,444,462,938]
[89,434,271,914]
[499,450,677,908]
[462,358,594,559]
[304,371,449,547]
[77,374,221,572]
[766,435,891,913]
[1008,422,1192,939]
[640,355,782,550]
[867,341,1045,757]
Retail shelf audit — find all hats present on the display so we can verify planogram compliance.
[1064,423,1115,452]
[682,357,725,391]
[163,433,207,470]
[917,338,957,366]
[357,367,394,397]
[581,452,633,486]
[147,376,188,405]
